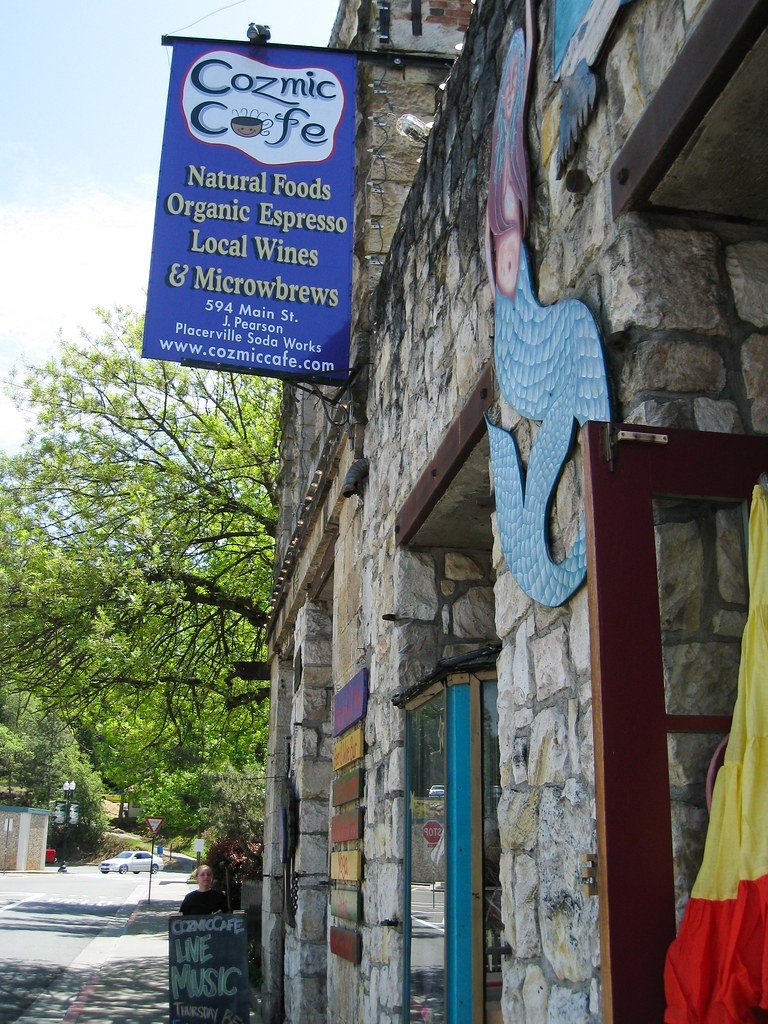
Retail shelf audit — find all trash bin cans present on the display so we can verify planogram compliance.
[157,846,163,854]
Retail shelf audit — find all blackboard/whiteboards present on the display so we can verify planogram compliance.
[168,912,250,1024]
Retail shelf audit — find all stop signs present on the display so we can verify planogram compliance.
[420,820,444,847]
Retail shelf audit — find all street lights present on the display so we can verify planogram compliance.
[58,781,77,873]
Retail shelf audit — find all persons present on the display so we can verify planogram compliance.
[179,864,229,915]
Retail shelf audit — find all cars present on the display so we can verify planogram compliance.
[426,785,445,800]
[100,789,129,803]
[97,850,164,874]
